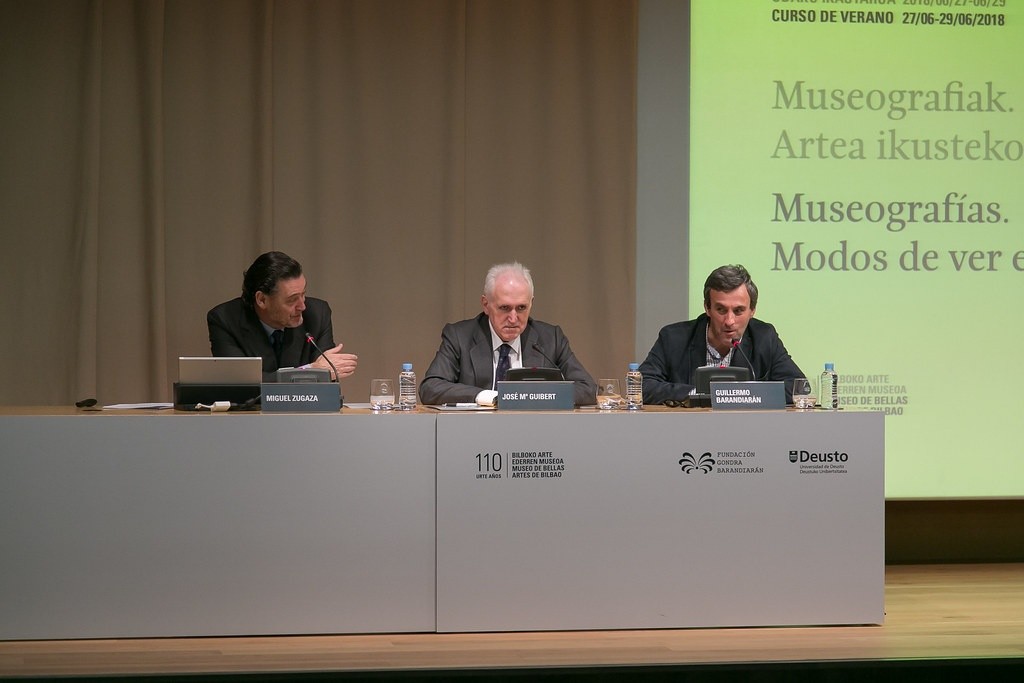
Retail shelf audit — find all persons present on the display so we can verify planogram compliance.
[207,251,357,382]
[640,264,811,407]
[418,262,600,408]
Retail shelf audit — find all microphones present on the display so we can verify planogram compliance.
[533,342,565,382]
[306,332,340,383]
[732,337,756,382]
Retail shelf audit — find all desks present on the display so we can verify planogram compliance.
[0,402,884,644]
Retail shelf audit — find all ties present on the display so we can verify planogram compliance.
[494,344,512,391]
[271,330,283,369]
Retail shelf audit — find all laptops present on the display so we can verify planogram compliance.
[178,357,263,386]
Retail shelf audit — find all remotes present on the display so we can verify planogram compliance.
[75,398,97,409]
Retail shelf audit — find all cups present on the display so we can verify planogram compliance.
[370,379,395,410]
[595,378,621,409]
[793,378,817,408]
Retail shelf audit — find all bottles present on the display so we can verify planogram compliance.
[821,363,837,409]
[625,363,643,409]
[398,364,417,411]
